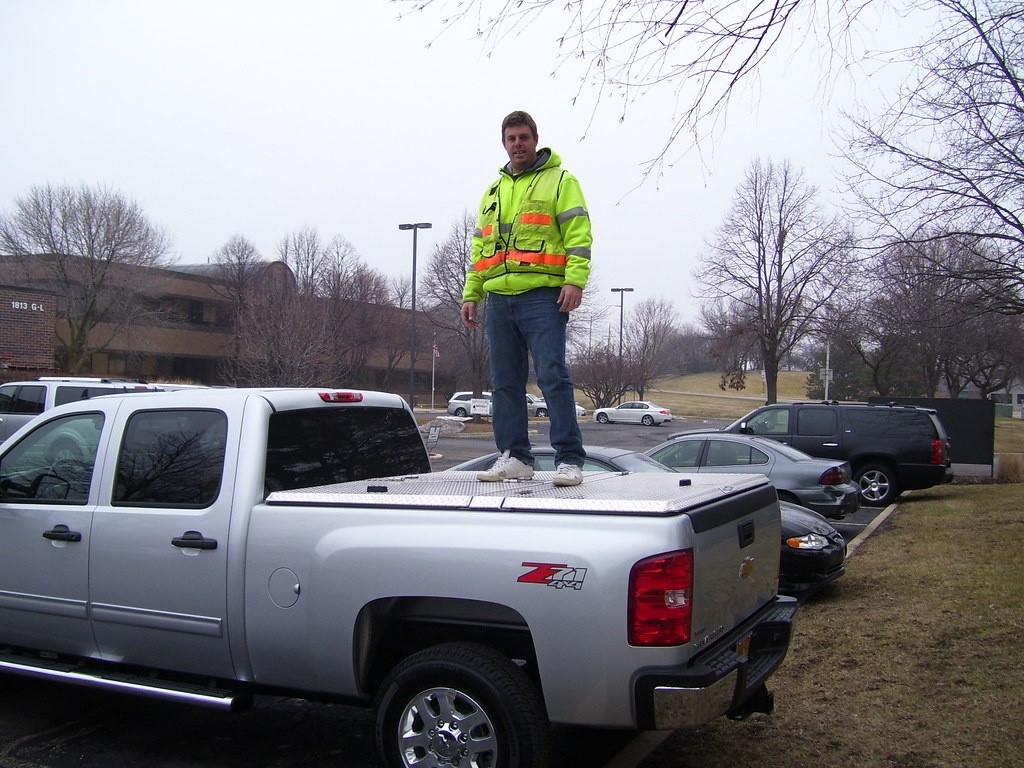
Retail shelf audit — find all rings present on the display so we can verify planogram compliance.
[575,303,580,305]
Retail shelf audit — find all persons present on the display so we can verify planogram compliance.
[461,111,593,487]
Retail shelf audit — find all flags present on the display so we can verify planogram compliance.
[433,345,440,357]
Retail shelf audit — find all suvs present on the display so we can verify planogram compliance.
[0,377,165,447]
[665,400,954,511]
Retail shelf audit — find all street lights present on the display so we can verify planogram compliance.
[611,288,634,405]
[399,223,433,413]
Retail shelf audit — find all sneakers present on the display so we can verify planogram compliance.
[552,463,583,485]
[476,449,534,481]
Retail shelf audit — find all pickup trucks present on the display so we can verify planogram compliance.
[0,390,800,768]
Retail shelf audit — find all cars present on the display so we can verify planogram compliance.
[447,392,493,418]
[538,397,587,417]
[439,445,847,588]
[640,432,861,521]
[592,401,675,426]
[525,393,549,418]
